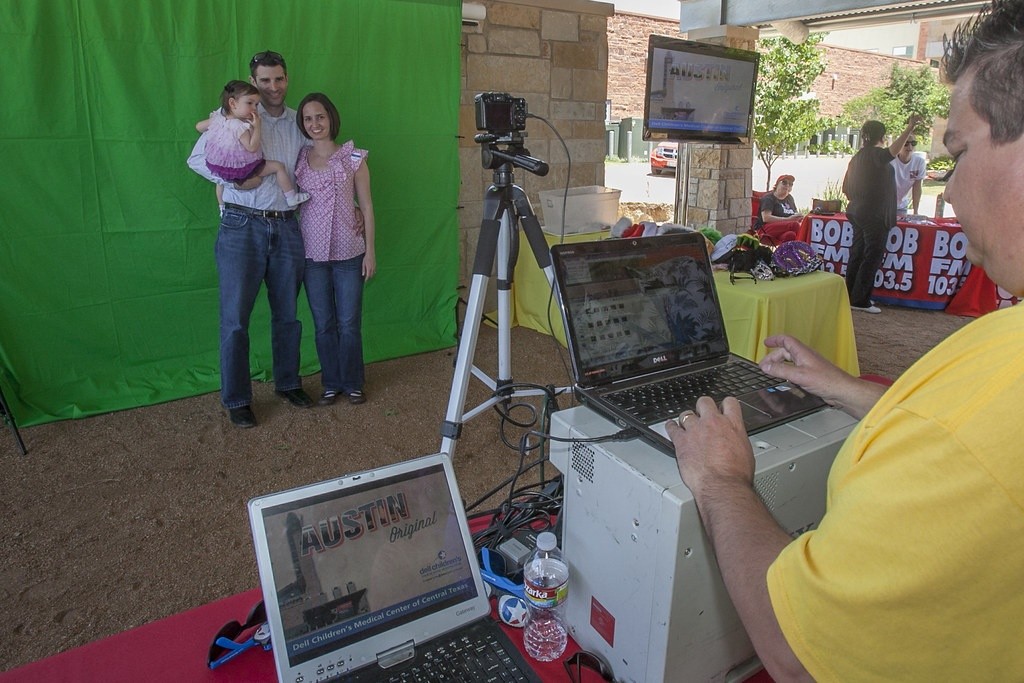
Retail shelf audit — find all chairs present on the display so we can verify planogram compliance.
[748,191,778,250]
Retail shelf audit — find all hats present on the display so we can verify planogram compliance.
[776,174,795,185]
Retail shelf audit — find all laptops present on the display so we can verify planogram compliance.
[549,233,827,460]
[246,451,546,683]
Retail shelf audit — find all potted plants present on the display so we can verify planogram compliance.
[813,179,848,215]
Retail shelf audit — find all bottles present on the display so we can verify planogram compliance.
[524,532,569,661]
[935,195,944,220]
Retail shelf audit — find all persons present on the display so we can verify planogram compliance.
[186,49,364,428]
[294,92,376,406]
[889,134,927,215]
[195,79,311,219]
[841,110,922,315]
[752,173,804,232]
[665,1,1023,681]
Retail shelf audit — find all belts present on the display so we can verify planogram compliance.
[225,203,293,218]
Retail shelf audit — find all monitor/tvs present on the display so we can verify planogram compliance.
[643,34,761,142]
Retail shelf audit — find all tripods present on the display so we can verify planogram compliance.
[437,132,589,463]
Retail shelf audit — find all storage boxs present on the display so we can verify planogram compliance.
[537,185,621,235]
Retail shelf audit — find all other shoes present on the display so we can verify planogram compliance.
[220,208,225,218]
[288,192,311,207]
[349,389,366,404]
[318,390,342,405]
[869,299,875,305]
[848,305,882,313]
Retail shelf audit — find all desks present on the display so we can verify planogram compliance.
[796,212,1024,318]
[483,229,862,379]
[0,501,775,683]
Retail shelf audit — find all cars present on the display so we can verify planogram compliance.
[651,141,678,175]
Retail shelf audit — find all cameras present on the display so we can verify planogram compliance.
[474,92,527,131]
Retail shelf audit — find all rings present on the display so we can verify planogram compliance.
[681,412,695,426]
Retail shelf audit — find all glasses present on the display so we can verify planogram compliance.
[904,140,918,146]
[206,600,267,670]
[477,547,525,600]
[253,52,282,63]
[483,580,530,627]
[780,180,793,187]
[563,651,617,683]
[253,621,272,651]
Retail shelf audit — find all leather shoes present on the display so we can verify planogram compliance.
[275,388,314,408]
[230,405,256,427]
